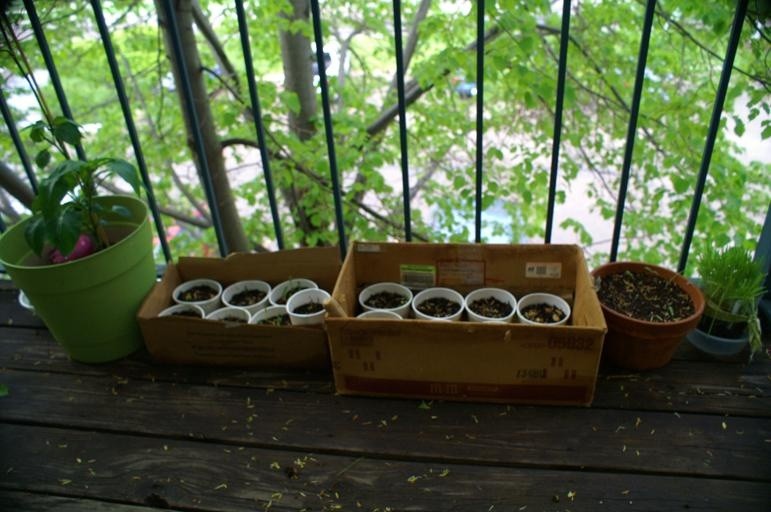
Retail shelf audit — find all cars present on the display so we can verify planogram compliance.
[449,63,478,99]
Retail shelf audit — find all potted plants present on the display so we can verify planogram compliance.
[1,112,158,365]
[687,243,771,363]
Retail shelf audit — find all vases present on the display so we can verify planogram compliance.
[593,263,701,365]
[412,287,465,319]
[286,289,331,326]
[465,287,516,322]
[157,278,318,326]
[518,293,571,326]
[358,282,413,319]
